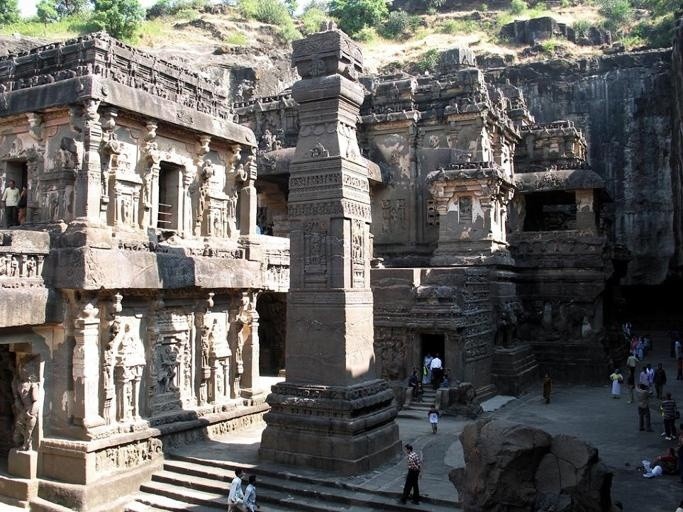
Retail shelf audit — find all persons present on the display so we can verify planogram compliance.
[227,468,246,512]
[409,351,448,403]
[0,180,22,228]
[242,474,261,512]
[256,222,274,236]
[427,405,440,434]
[394,444,423,505]
[609,317,683,477]
[543,372,553,404]
[676,500,683,512]
[18,183,27,227]
